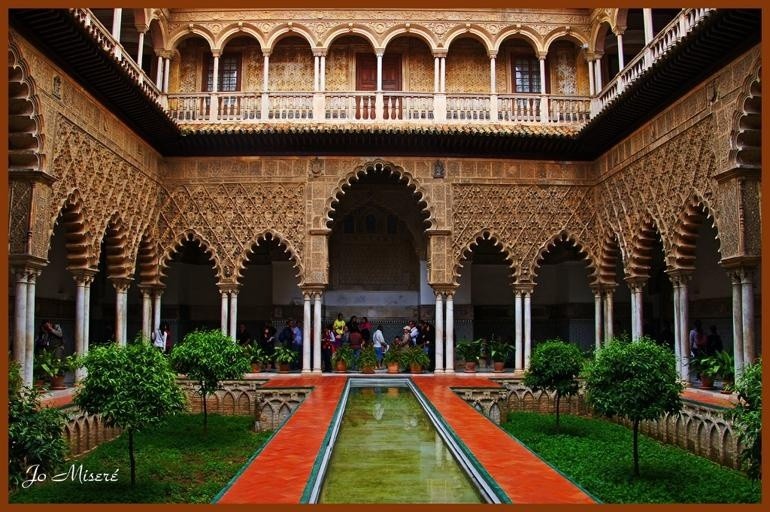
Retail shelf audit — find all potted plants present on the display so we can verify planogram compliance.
[685,352,719,386]
[713,348,738,390]
[242,338,517,373]
[34,355,46,388]
[39,348,79,387]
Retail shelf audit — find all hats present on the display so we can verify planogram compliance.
[402,325,411,331]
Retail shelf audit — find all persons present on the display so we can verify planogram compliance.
[236,322,250,346]
[165,323,172,352]
[41,319,65,376]
[347,402,410,429]
[643,320,723,381]
[152,324,167,353]
[260,314,435,372]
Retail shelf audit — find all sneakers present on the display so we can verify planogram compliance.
[378,367,384,370]
